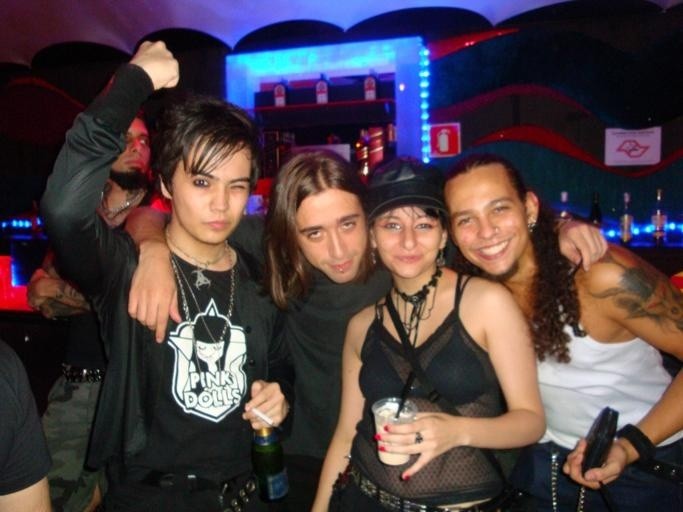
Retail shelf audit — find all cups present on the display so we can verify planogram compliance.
[373,398,421,467]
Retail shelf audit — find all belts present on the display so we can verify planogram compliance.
[60,368,104,382]
[117,463,260,512]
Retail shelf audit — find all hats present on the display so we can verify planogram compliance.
[366,155,449,219]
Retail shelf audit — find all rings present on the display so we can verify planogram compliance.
[415,430,422,444]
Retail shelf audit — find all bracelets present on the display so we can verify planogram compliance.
[617,423,657,462]
[557,217,570,235]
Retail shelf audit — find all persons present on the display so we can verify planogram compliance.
[39,39,284,511]
[25,109,152,512]
[443,154,683,511]
[309,154,547,510]
[126,148,608,509]
[0,337,52,512]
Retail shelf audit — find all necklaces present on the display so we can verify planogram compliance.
[102,181,139,220]
[391,262,439,351]
[169,237,238,394]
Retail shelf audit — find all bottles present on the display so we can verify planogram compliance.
[250,429,290,503]
[620,192,634,245]
[362,66,379,102]
[652,189,667,240]
[316,72,331,103]
[591,193,600,230]
[557,191,571,220]
[271,77,289,104]
